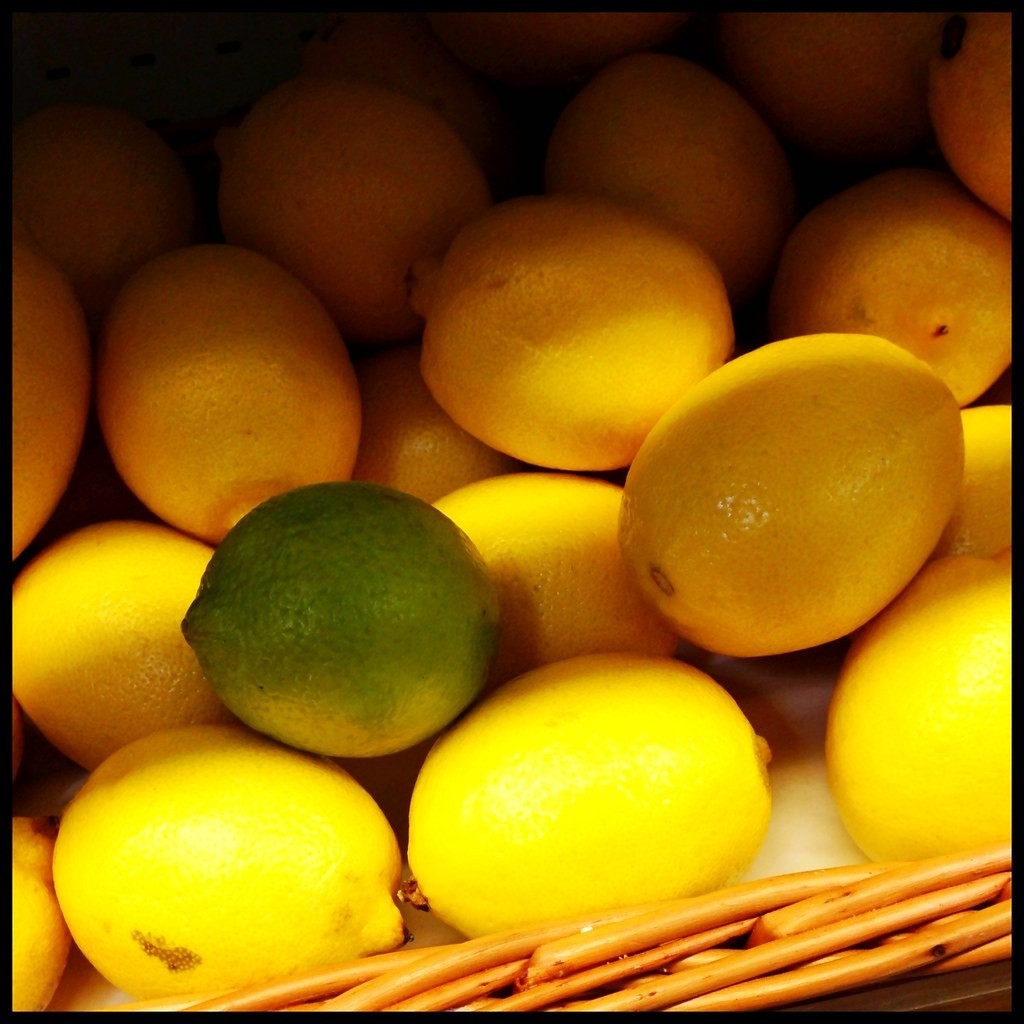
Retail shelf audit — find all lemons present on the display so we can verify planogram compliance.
[11,11,1014,1015]
